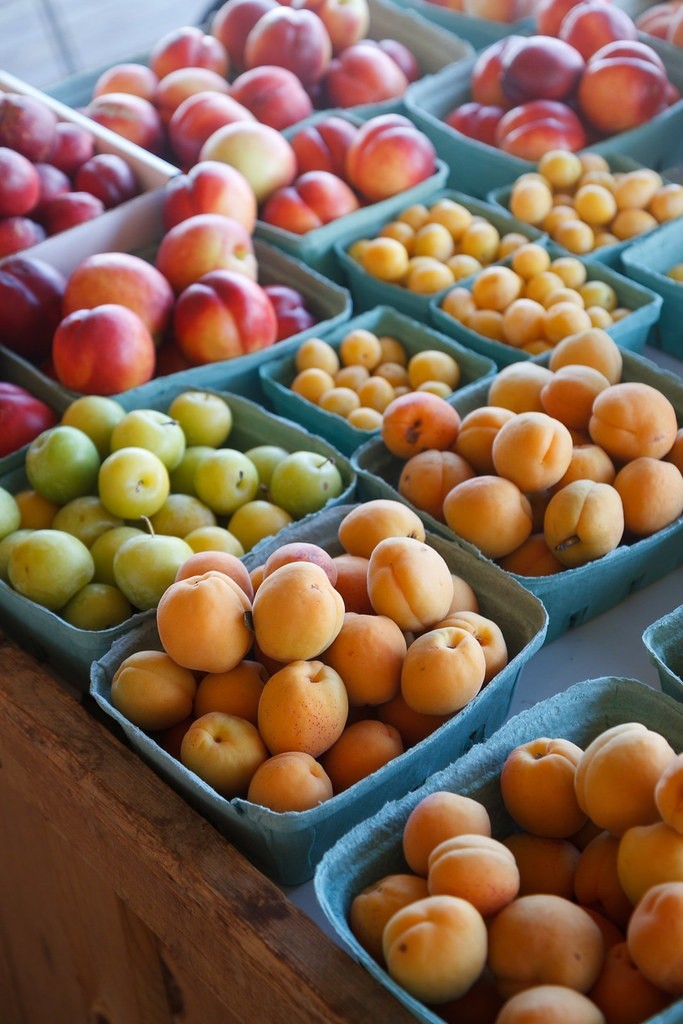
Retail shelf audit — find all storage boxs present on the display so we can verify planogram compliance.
[0,0,683,1024]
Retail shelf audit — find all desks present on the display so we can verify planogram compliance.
[0,624,424,1024]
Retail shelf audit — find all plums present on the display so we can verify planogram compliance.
[0,1,683,458]
